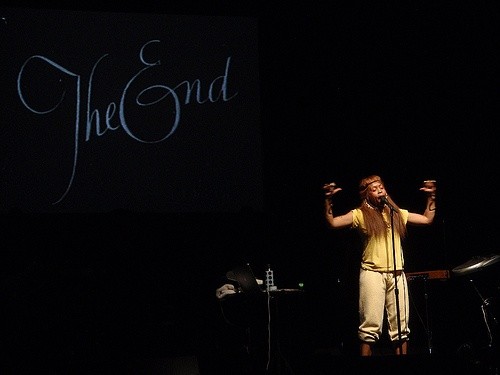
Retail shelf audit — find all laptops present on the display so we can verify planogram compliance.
[235,262,305,293]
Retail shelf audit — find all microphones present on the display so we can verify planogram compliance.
[381,197,399,213]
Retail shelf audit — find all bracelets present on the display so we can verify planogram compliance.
[426,194,437,211]
[324,197,333,216]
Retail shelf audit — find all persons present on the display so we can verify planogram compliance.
[322,175,438,356]
